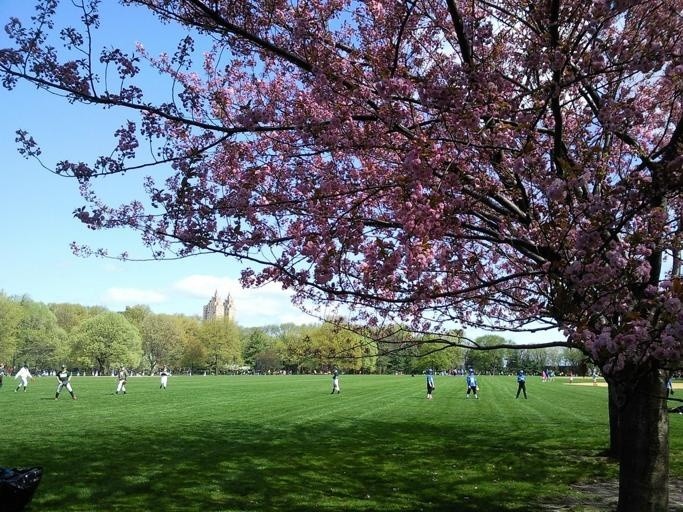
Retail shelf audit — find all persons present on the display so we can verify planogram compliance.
[667,379,673,399]
[55,365,77,400]
[160,367,167,389]
[467,369,479,398]
[331,369,340,394]
[542,368,555,382]
[0,363,4,385]
[570,375,573,383]
[427,369,435,399]
[14,365,32,392]
[448,368,464,376]
[515,370,527,399]
[4,365,13,377]
[593,373,598,386]
[116,366,128,394]
